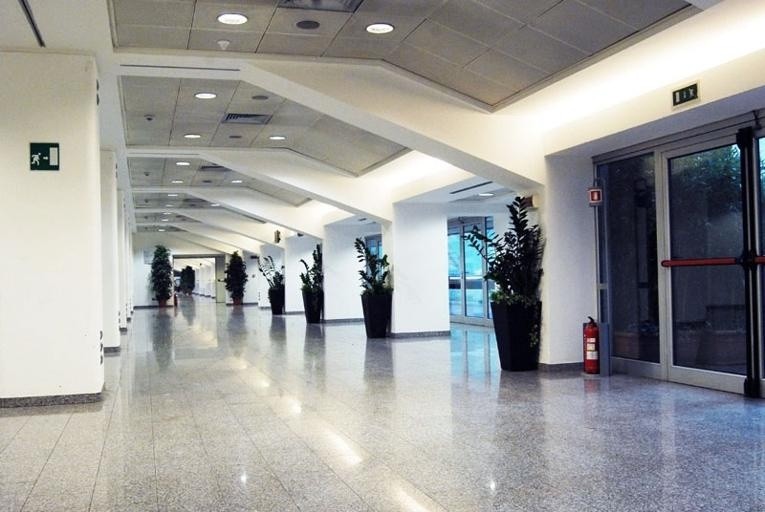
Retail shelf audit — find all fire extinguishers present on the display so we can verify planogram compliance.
[583,317,600,375]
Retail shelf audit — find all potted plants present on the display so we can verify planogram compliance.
[354,237,394,338]
[460,196,544,371]
[257,255,284,314]
[298,244,323,323]
[151,243,195,307]
[217,250,249,305]
[614,312,746,366]
